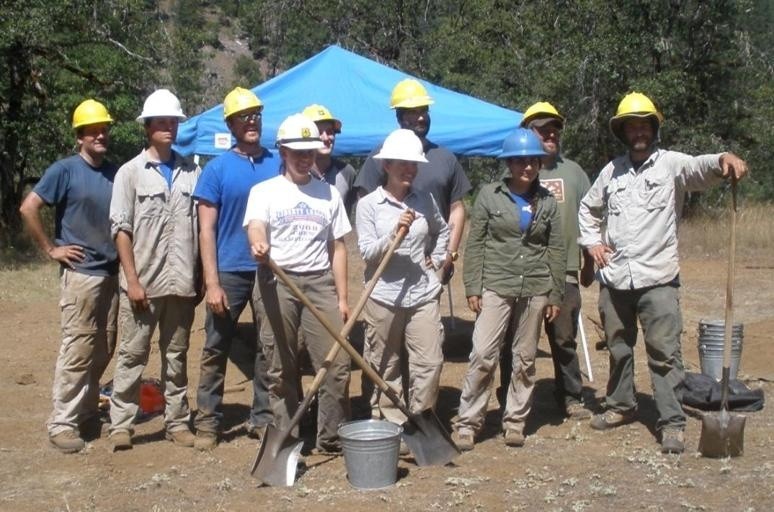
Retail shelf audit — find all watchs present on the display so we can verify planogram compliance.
[446,249,458,261]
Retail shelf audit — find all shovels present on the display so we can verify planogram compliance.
[250,208,415,487]
[260,248,461,470]
[699,167,747,458]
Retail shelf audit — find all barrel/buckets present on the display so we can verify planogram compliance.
[698,320,744,380]
[337,419,405,488]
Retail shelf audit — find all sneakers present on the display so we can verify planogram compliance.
[660,428,685,453]
[589,408,638,430]
[503,428,525,446]
[48,417,266,452]
[451,422,475,451]
[552,390,594,419]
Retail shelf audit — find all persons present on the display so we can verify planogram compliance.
[109,89,205,451]
[577,92,748,455]
[301,103,357,379]
[499,102,591,419]
[451,128,567,451]
[188,86,286,451]
[356,129,451,455]
[19,99,120,453]
[353,78,471,404]
[242,113,352,454]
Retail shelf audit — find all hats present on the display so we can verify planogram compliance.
[528,117,564,129]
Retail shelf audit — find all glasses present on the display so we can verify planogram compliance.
[239,112,262,122]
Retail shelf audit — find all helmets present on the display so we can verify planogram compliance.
[135,89,187,125]
[608,91,662,147]
[520,101,564,130]
[390,79,434,109]
[72,98,114,129]
[275,115,325,150]
[302,104,342,133]
[223,86,264,121]
[372,128,429,163]
[496,127,550,162]
